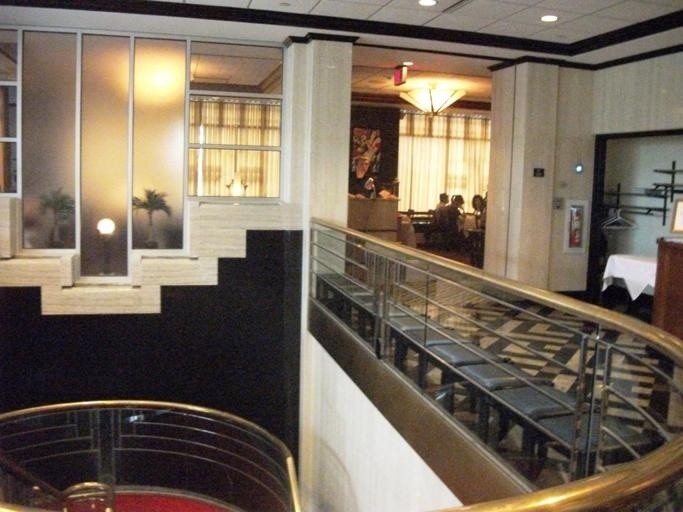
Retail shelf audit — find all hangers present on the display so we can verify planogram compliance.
[596,208,639,230]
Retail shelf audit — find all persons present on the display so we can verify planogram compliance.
[433,191,486,250]
[348,155,377,201]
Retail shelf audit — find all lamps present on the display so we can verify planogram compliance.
[399,87,467,115]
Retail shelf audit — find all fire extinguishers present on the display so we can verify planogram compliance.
[570,210,583,247]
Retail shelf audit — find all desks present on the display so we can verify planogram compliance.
[602,253,657,316]
